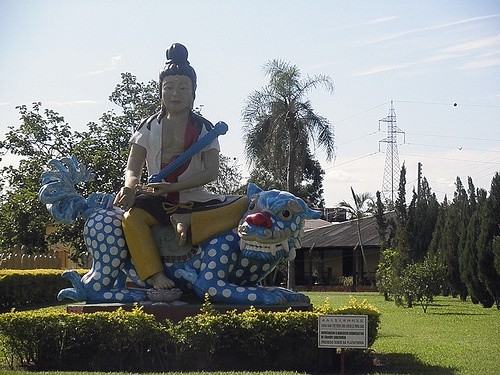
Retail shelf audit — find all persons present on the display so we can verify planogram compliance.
[113,43,250,291]
[312,266,319,284]
